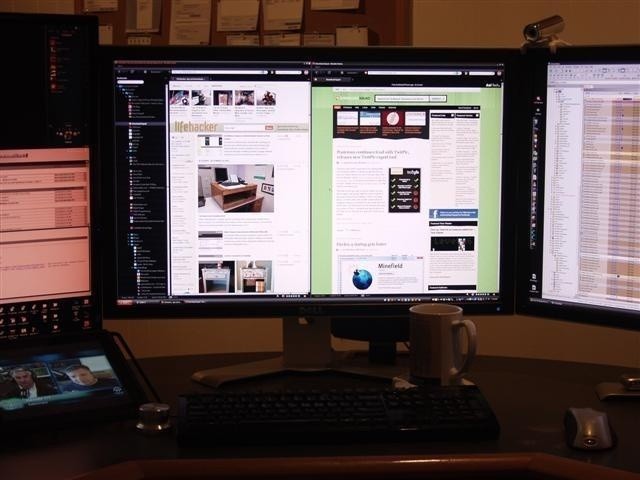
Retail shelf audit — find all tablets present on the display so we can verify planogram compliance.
[1,328,146,430]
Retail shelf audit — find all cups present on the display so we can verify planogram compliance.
[409,304,478,378]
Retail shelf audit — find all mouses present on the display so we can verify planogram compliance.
[565,406,618,452]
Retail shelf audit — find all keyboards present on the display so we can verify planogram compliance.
[177,384,500,444]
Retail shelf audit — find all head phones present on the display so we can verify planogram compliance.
[10,367,38,385]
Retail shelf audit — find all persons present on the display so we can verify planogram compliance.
[3,368,57,406]
[60,363,117,403]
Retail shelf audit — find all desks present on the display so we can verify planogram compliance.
[0,351,639,480]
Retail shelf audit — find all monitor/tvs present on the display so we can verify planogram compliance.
[0,12,103,342]
[100,45,514,388]
[514,44,640,402]
